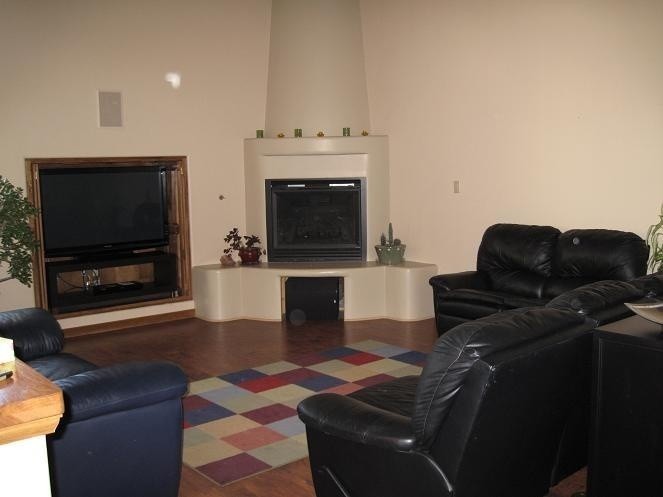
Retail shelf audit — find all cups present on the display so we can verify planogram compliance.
[294,129,302,137]
[256,129,263,138]
[343,128,351,136]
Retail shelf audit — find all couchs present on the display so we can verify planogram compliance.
[296,269,662,493]
[425,223,654,343]
[0,305,187,496]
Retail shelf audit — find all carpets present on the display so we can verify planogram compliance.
[181,336,430,486]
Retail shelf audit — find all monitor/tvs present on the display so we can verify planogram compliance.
[265,177,368,263]
[38,165,170,264]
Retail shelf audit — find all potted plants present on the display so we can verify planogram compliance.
[374,222,407,265]
[223,226,266,265]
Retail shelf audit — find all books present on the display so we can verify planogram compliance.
[0,371,13,383]
[0,336,14,363]
[0,363,16,374]
